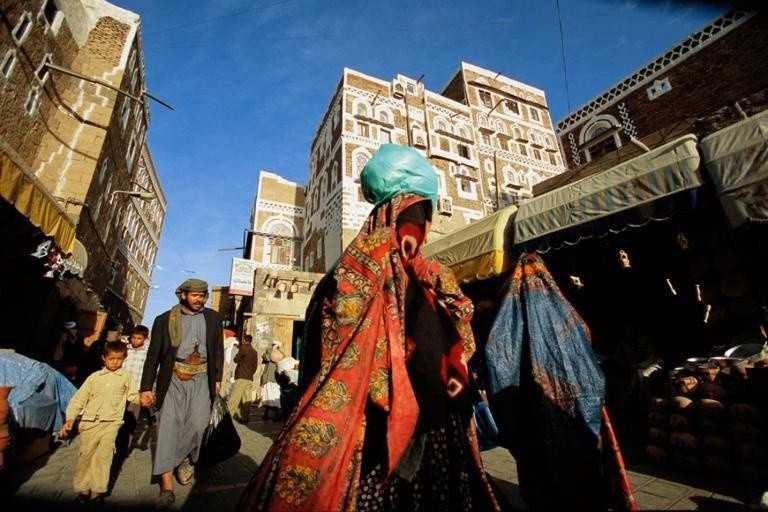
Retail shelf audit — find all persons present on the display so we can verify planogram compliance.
[485,252,639,512]
[60,279,224,508]
[262,341,286,402]
[227,335,258,416]
[234,184,515,512]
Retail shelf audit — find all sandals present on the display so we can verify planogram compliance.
[152,490,174,511]
[177,458,195,484]
[72,494,101,506]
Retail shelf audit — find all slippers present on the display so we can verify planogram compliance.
[236,418,248,423]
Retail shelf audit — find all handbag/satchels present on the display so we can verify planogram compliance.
[198,393,242,466]
